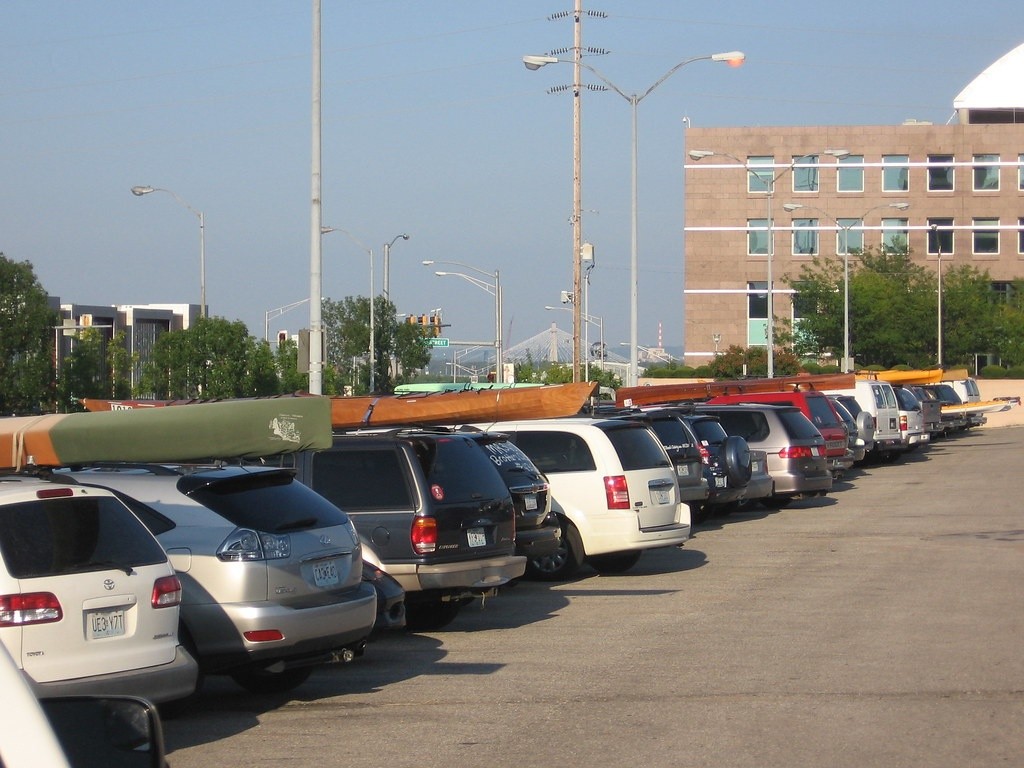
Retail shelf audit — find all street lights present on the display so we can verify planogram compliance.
[523,52,747,390]
[690,149,851,378]
[931,223,943,368]
[620,342,671,372]
[782,202,909,375]
[545,305,604,374]
[421,260,501,383]
[384,233,410,313]
[434,270,503,382]
[320,225,375,395]
[130,185,208,405]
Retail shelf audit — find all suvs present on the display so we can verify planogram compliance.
[0,473,200,712]
[421,424,561,565]
[24,460,380,720]
[246,428,526,641]
[445,377,1022,585]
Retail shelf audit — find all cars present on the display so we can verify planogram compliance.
[0,641,168,768]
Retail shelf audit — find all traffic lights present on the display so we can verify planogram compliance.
[417,315,427,337]
[406,316,414,326]
[430,316,439,338]
